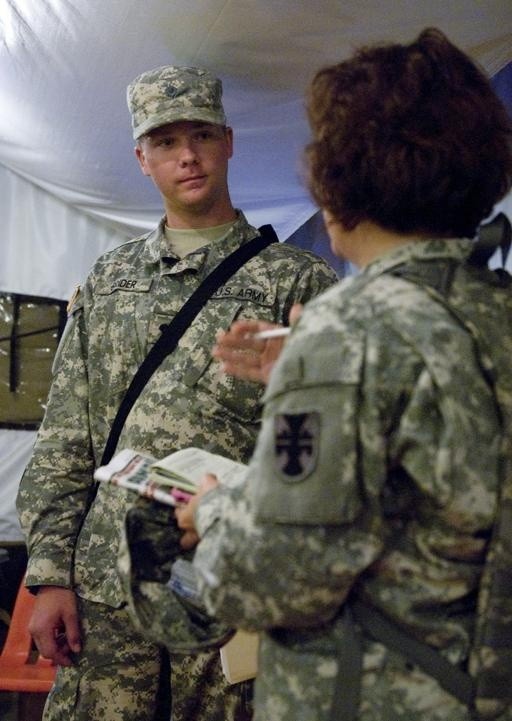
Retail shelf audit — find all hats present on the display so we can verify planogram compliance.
[126,66,226,140]
[118,497,235,651]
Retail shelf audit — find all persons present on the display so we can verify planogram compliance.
[15,65,342,721]
[174,26,512,721]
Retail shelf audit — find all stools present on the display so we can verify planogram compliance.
[0,576,59,694]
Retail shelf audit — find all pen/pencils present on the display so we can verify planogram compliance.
[253,326,292,340]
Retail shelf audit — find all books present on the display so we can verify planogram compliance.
[146,446,250,496]
